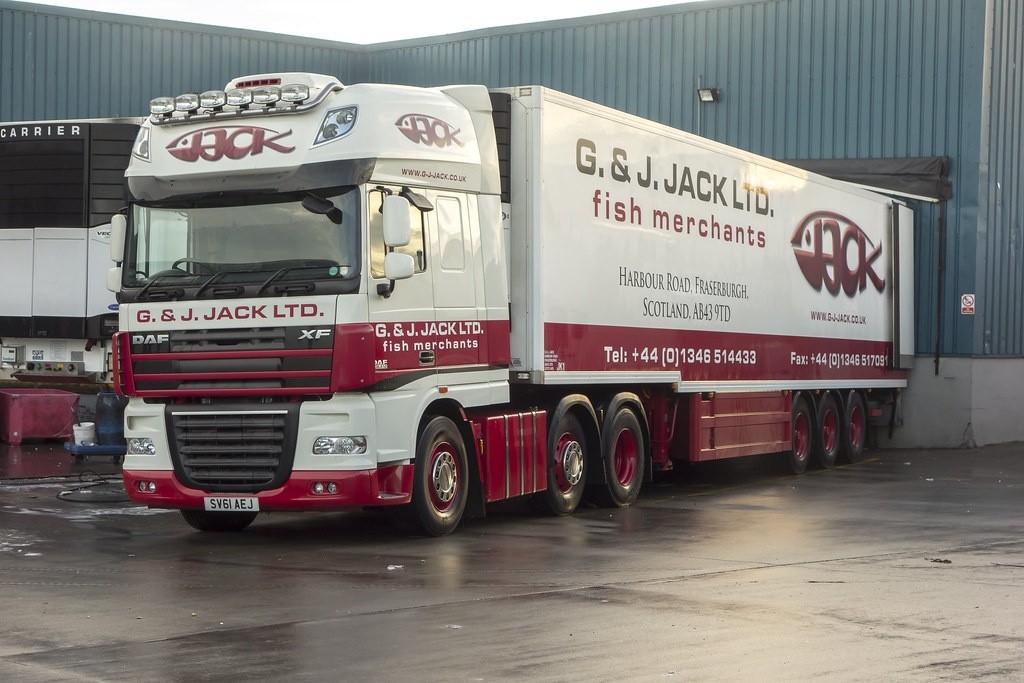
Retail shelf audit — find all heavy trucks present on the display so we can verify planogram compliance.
[105,73,916,537]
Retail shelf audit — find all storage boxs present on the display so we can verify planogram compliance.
[0,388,80,446]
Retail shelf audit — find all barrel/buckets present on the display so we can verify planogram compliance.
[72,422,95,445]
[95,391,125,445]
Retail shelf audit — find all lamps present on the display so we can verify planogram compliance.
[698,87,720,102]
